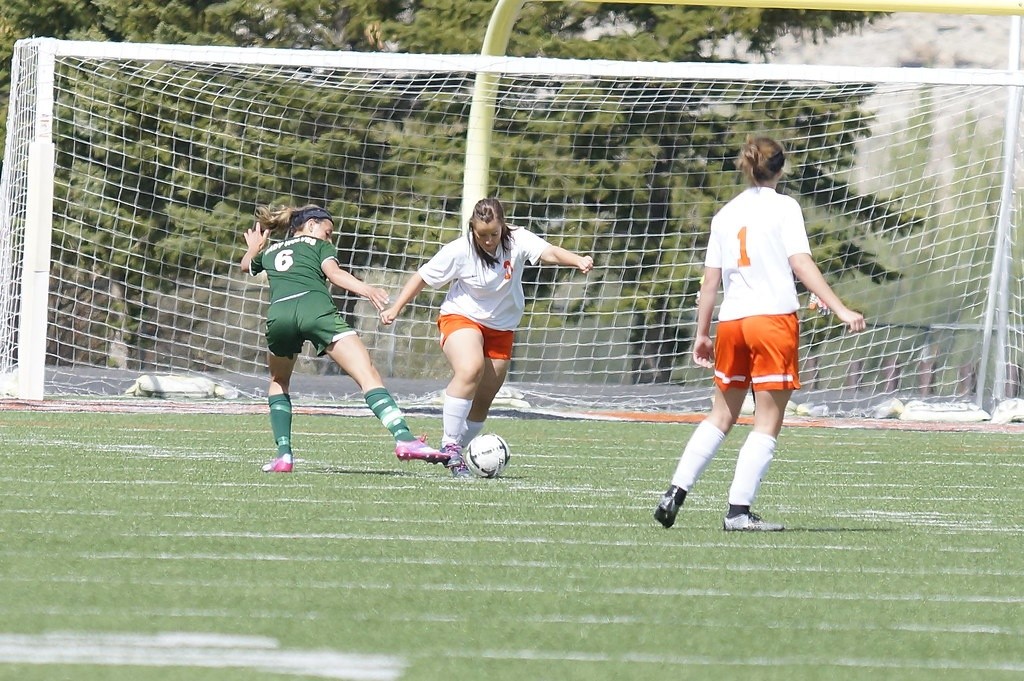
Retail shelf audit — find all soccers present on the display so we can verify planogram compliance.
[465,433,511,478]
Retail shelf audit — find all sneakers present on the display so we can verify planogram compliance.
[440,443,473,480]
[261,452,294,474]
[723,509,786,533]
[653,494,679,528]
[395,437,451,464]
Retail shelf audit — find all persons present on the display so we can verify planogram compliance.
[655,136,867,532]
[380,198,593,479]
[240,203,451,472]
